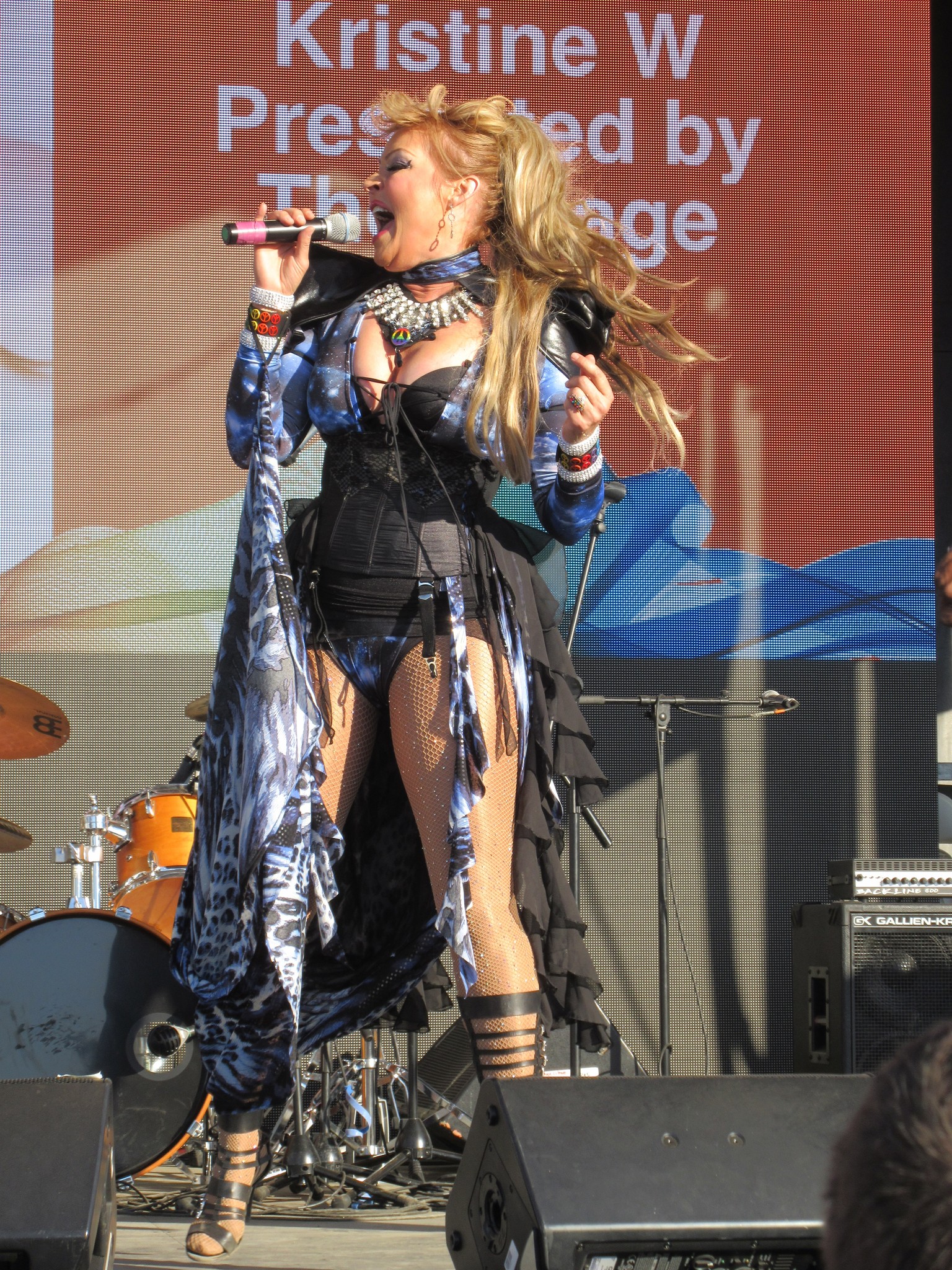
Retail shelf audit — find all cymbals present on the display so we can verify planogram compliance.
[0,816,33,857]
[0,677,71,762]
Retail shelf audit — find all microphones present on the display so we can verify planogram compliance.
[221,212,361,245]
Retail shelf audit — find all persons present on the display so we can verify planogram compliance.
[822,1018,952,1270]
[186,81,731,1262]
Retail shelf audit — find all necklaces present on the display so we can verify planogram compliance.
[363,280,486,368]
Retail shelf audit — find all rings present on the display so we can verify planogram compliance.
[569,394,588,411]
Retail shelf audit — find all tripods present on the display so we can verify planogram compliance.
[331,1035,464,1211]
[176,1041,451,1214]
[251,1056,423,1212]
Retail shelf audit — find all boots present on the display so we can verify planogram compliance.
[451,987,553,1087]
[177,1004,293,1264]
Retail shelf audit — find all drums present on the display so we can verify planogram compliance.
[103,783,197,941]
[0,906,213,1187]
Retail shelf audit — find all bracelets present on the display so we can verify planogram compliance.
[557,424,603,484]
[237,284,295,355]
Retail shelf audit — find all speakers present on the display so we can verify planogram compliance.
[790,903,951,1077]
[437,1068,878,1270]
[0,1078,117,1269]
